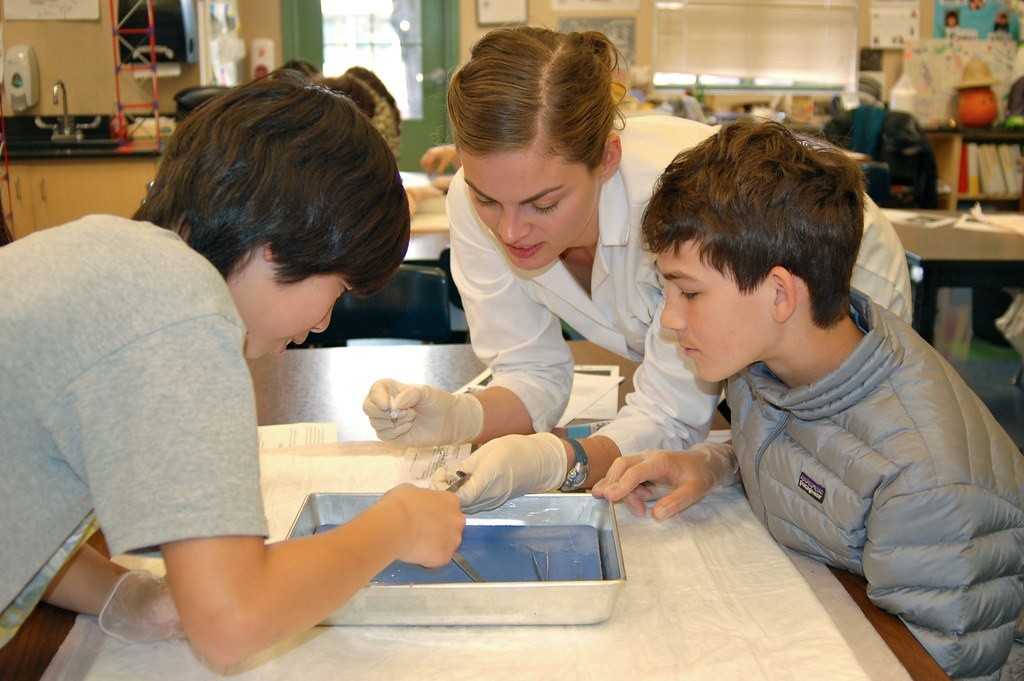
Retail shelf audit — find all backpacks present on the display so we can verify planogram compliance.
[822,106,940,212]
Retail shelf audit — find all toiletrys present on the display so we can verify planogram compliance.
[2,43,40,113]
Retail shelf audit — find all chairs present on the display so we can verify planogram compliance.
[282,264,450,349]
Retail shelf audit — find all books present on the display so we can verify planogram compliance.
[959,143,1023,194]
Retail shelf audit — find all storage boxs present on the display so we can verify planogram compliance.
[288,491,631,625]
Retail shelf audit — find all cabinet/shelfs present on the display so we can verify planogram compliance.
[789,125,1024,213]
[649,93,832,124]
[0,115,160,241]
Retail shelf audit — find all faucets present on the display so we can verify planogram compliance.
[34,79,102,144]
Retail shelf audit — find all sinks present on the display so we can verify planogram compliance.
[23,139,121,152]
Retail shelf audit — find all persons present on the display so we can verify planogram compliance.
[590,115,1024,681]
[364,28,913,519]
[995,14,1011,32]
[944,12,959,28]
[0,58,462,667]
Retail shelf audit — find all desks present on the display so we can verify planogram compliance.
[0,340,956,681]
[883,207,1024,349]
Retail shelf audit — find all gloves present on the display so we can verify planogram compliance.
[425,432,568,515]
[362,378,483,450]
[98,569,188,644]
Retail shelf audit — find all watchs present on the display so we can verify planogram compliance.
[559,438,589,492]
